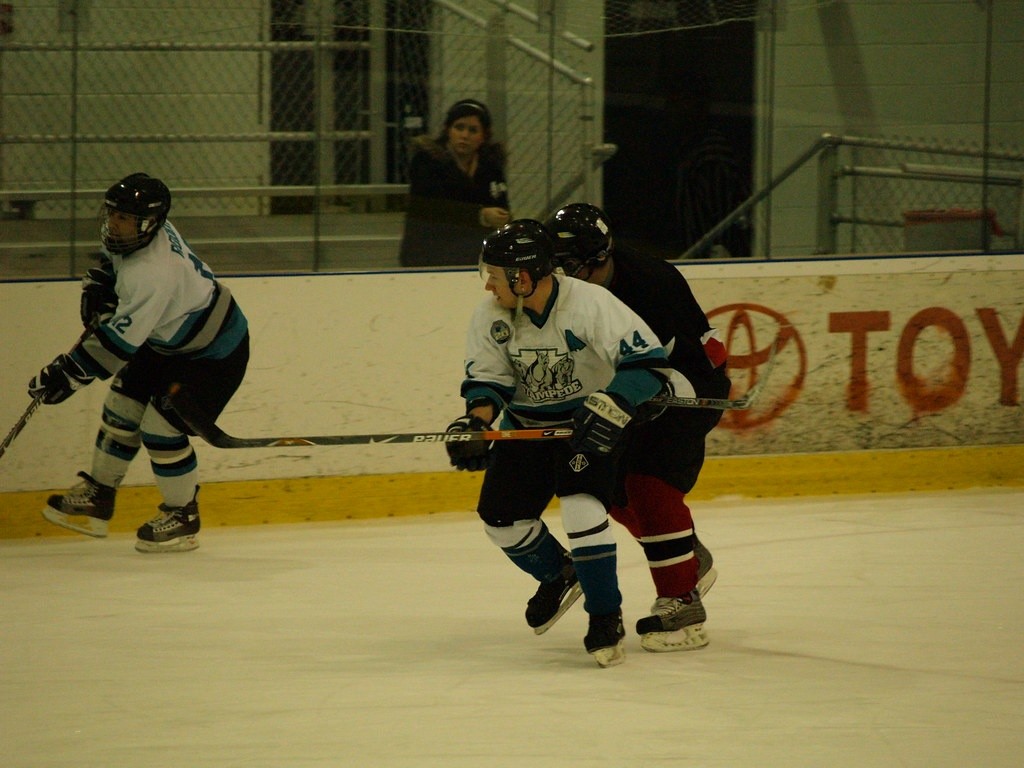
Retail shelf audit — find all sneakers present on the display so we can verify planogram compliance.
[42,470,117,540]
[525,551,586,635]
[636,588,710,653]
[135,485,201,553]
[583,597,626,667]
[692,536,718,599]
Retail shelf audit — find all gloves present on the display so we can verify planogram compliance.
[635,381,675,427]
[445,413,495,472]
[81,268,118,330]
[27,353,96,405]
[569,389,635,457]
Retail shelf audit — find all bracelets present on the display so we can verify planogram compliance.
[479,207,493,228]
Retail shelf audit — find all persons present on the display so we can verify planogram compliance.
[445,202,732,666]
[27,172,251,553]
[397,97,513,265]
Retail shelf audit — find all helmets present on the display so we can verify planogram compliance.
[481,219,555,284]
[101,172,172,255]
[552,203,613,266]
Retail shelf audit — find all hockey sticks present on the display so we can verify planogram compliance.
[1,381,53,459]
[640,337,778,410]
[147,357,567,449]
